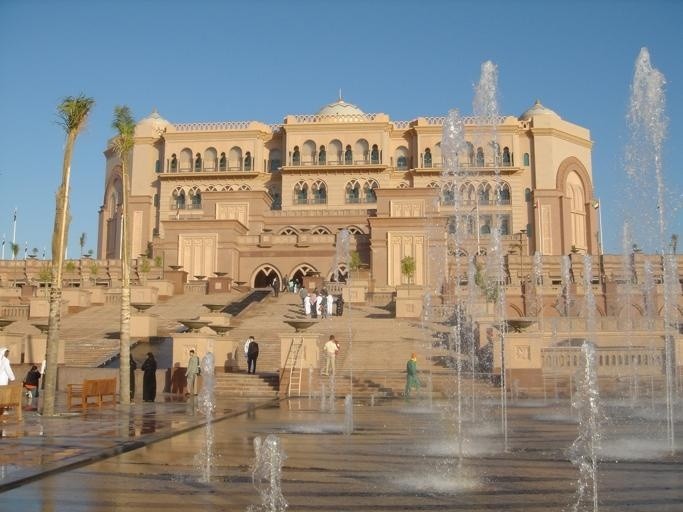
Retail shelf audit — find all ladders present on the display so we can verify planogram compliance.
[287,337,305,397]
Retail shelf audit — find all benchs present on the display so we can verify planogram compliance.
[0,382,23,425]
[67,377,117,410]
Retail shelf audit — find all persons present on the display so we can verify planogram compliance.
[184,350,199,396]
[270,273,345,319]
[23,365,41,412]
[0,346,15,385]
[140,352,156,402]
[246,336,258,374]
[244,338,254,372]
[322,335,339,375]
[129,353,136,406]
[403,352,421,404]
